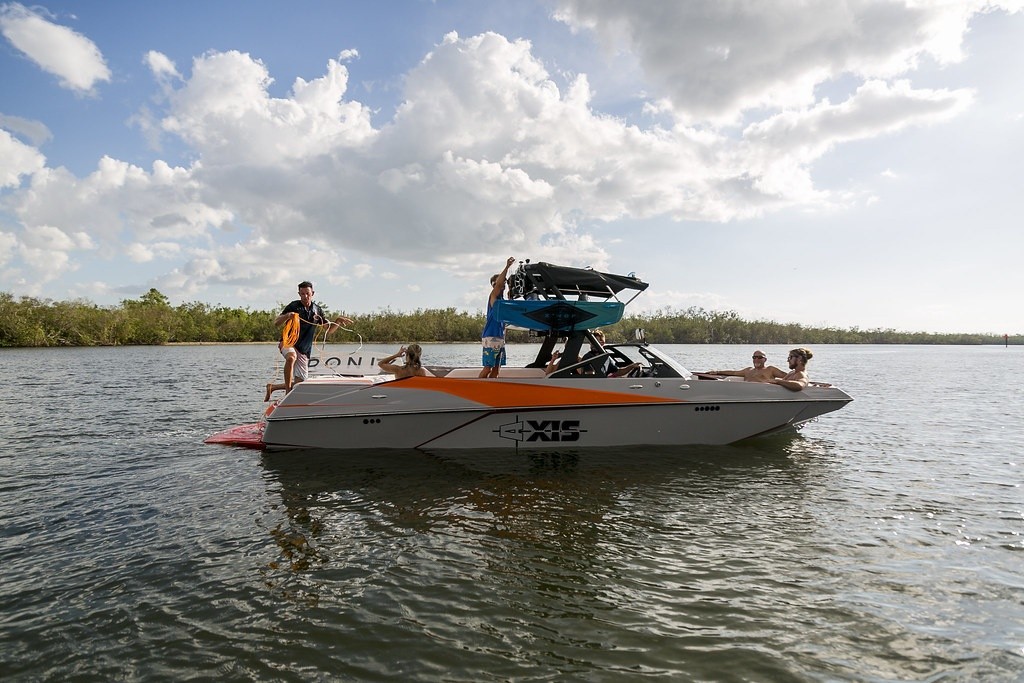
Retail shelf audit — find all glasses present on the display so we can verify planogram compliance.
[788,356,797,362]
[752,356,763,359]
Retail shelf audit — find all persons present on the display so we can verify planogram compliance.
[766,347,813,390]
[545,338,582,375]
[378,344,426,380]
[705,350,788,383]
[581,329,645,377]
[478,256,515,378]
[263,281,353,403]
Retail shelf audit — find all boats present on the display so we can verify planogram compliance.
[204,262,854,450]
